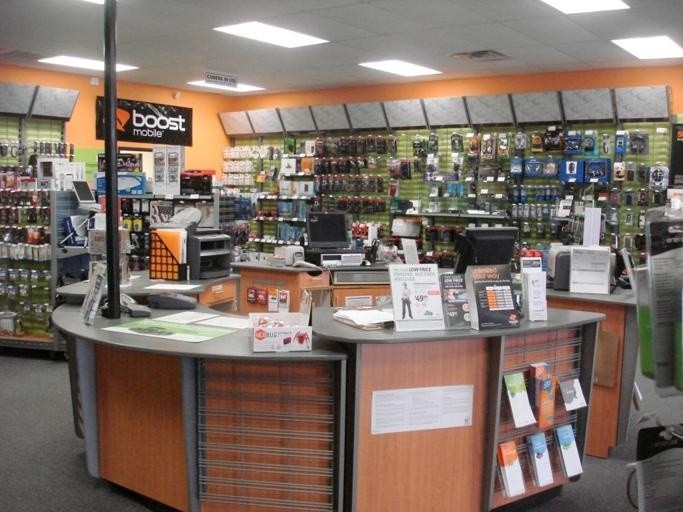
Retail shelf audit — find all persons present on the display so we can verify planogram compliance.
[401,283,413,319]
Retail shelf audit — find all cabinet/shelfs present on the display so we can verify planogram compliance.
[0,189,98,353]
[121,195,220,272]
[221,123,669,267]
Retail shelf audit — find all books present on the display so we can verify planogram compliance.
[466,263,520,330]
[152,229,187,281]
[387,264,446,332]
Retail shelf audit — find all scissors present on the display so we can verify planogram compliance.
[370,239,379,253]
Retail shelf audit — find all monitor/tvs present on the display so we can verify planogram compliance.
[305,211,350,250]
[452,226,520,275]
